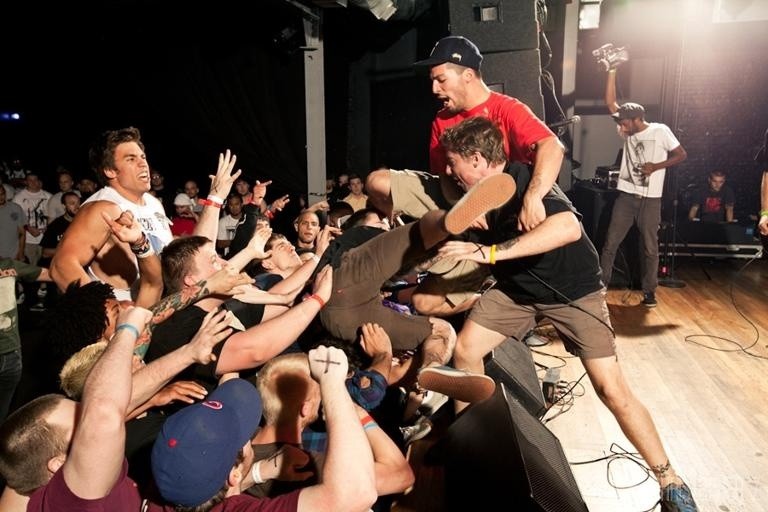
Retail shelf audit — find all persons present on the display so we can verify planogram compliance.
[687,169,735,222]
[752,129,768,238]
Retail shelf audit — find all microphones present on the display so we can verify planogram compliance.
[547,115,580,128]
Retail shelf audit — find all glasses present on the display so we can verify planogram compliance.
[151,175,162,181]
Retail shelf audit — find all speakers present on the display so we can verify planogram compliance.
[575,181,643,288]
[440,383,588,512]
[427,329,547,427]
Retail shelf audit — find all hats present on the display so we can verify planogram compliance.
[146,373,269,511]
[406,33,487,75]
[607,100,648,126]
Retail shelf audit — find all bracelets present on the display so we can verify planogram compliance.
[759,211,768,216]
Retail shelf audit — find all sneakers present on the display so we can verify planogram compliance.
[655,470,702,512]
[640,291,660,310]
[415,360,498,407]
[440,170,520,237]
